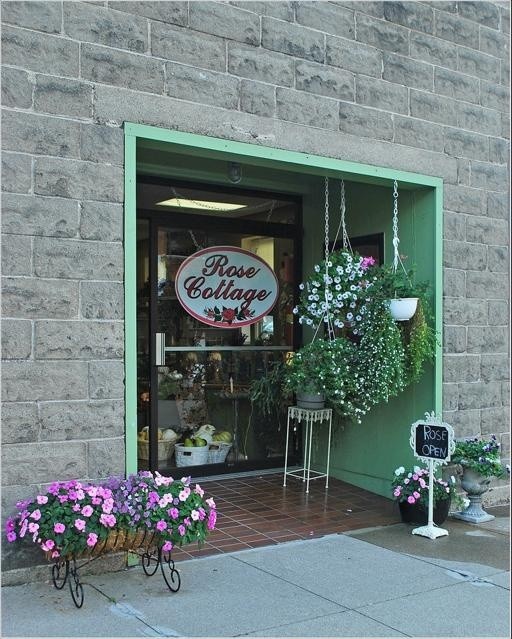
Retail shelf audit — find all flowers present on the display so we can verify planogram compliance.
[393,466,463,509]
[5,469,218,561]
[242,246,443,461]
[450,436,511,481]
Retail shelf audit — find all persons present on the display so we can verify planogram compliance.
[174,352,233,429]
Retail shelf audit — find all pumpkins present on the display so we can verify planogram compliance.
[200,425,216,435]
[213,432,232,442]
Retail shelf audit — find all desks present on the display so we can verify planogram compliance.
[283,406,333,494]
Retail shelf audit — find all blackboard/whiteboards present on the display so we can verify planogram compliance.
[416,424,448,459]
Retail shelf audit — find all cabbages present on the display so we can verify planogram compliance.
[140,426,176,440]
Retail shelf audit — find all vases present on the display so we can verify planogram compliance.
[397,497,451,527]
[452,466,496,523]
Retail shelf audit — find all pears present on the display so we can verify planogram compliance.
[184,438,205,447]
